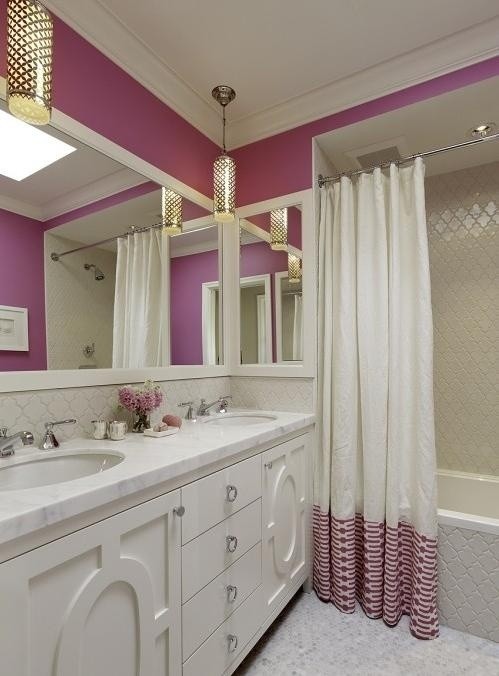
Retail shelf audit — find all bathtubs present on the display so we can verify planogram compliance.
[436,468,499,645]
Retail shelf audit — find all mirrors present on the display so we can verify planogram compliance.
[218,185,321,384]
[154,210,223,366]
[0,72,232,395]
[238,217,302,366]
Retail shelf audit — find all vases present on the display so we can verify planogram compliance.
[129,408,152,433]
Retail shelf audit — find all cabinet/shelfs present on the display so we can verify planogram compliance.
[261,424,310,647]
[169,445,264,675]
[0,489,184,675]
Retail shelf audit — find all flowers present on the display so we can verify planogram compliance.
[114,375,162,430]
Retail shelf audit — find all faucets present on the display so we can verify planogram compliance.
[196,398,229,416]
[0,428,34,458]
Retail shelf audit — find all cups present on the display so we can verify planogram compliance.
[90,420,128,441]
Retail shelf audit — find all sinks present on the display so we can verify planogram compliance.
[203,412,278,426]
[0,448,126,492]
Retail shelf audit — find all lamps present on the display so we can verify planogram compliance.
[266,207,292,251]
[285,253,301,284]
[159,185,183,236]
[4,0,57,126]
[208,81,241,221]
[84,263,105,281]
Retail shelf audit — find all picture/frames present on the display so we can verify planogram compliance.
[0,304,29,352]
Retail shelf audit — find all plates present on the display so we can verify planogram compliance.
[144,425,178,439]
